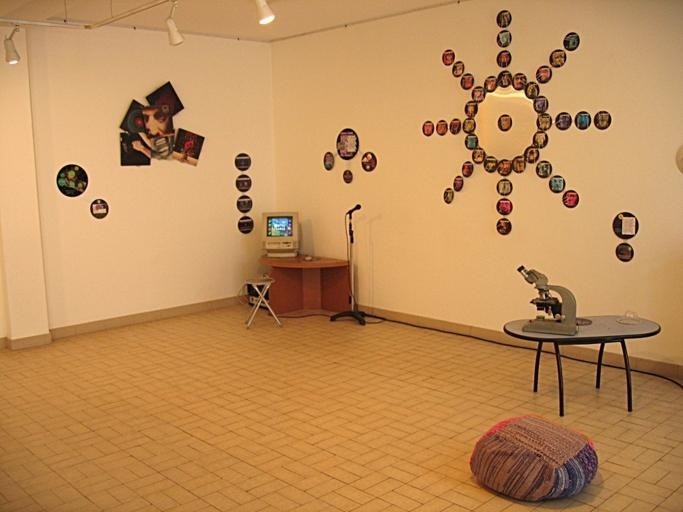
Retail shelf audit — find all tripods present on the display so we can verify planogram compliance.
[330,216,366,326]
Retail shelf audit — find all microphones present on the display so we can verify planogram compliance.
[345,204,361,215]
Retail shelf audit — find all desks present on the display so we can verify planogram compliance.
[503,314,659,416]
[258,252,351,316]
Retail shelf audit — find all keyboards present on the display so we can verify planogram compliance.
[267,252,297,257]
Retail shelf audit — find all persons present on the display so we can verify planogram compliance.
[142,105,171,138]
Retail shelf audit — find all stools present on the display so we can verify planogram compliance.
[243,278,283,329]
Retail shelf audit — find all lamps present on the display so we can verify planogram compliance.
[166,1,276,48]
[4,23,22,65]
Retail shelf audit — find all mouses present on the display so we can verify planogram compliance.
[305,256,313,261]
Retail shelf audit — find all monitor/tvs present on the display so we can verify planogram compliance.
[263,212,298,249]
